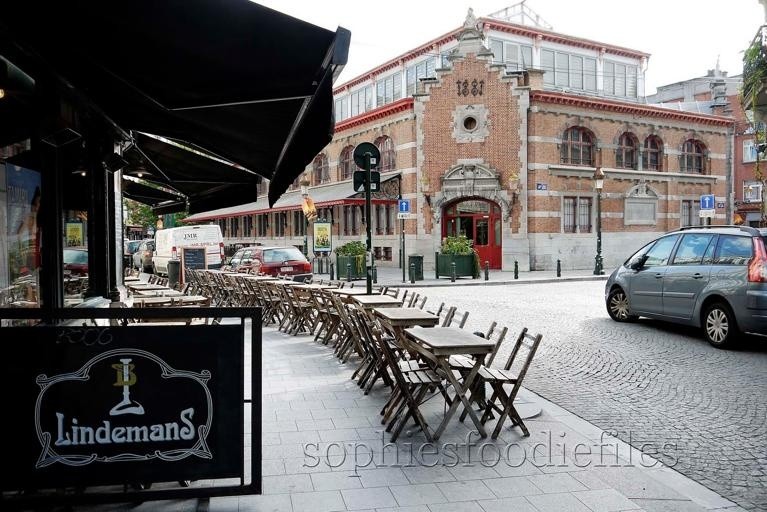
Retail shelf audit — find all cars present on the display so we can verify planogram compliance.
[124,239,155,272]
[62,245,88,276]
[605,223,767,349]
[220,245,313,284]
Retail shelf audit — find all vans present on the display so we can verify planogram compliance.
[152,225,225,275]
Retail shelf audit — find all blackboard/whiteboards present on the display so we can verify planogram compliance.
[181,246,208,269]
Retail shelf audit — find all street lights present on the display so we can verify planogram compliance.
[592,166,605,275]
[299,172,310,258]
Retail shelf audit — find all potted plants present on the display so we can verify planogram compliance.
[332,241,370,280]
[435,234,481,279]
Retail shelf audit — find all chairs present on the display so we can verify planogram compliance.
[0,267,230,327]
[183,268,544,443]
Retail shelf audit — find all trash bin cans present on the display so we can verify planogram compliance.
[408,255,424,281]
[167,260,180,284]
